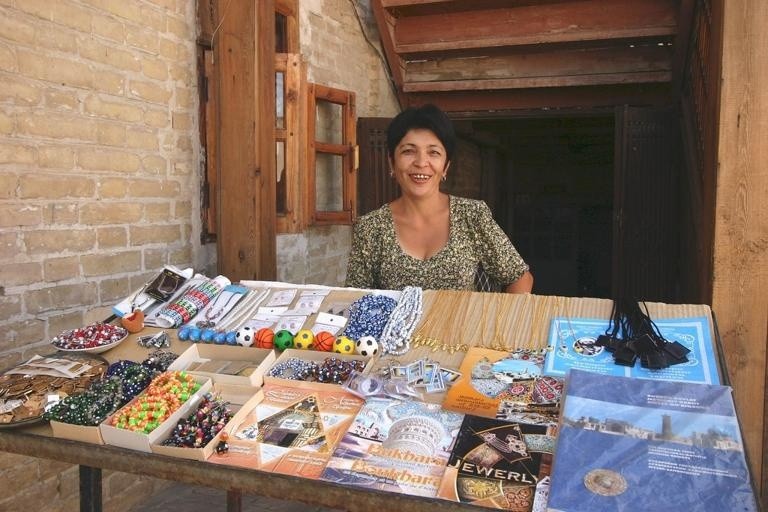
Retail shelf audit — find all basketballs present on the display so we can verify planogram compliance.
[314,332,335,351]
[255,328,273,348]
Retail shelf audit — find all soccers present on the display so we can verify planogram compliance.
[235,326,255,347]
[294,329,314,350]
[273,330,294,352]
[355,336,378,357]
[333,336,355,354]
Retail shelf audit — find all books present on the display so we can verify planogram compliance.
[208,384,366,478]
[320,397,466,499]
[530,368,760,512]
[437,414,547,511]
[546,317,719,388]
[442,347,566,427]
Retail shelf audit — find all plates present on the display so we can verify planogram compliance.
[0,350,111,429]
[49,323,129,354]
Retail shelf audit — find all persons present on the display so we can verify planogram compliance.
[344,104,534,293]
